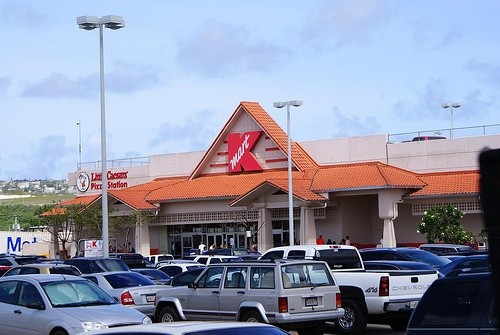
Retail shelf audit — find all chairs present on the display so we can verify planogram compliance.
[228,273,247,287]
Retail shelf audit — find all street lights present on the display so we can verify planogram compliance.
[441,102,461,138]
[75,12,126,261]
[273,99,304,245]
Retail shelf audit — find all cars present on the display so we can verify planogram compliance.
[84,271,160,318]
[355,242,490,276]
[0,263,84,295]
[0,246,261,282]
[80,319,292,335]
[0,274,152,335]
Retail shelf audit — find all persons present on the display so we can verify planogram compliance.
[342,239,346,245]
[316,235,324,245]
[128,242,135,253]
[108,245,114,253]
[171,241,175,257]
[379,235,383,248]
[273,240,281,248]
[199,241,207,255]
[327,239,332,244]
[78,174,88,187]
[210,244,215,249]
[345,236,350,246]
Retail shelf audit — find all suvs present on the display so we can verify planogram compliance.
[154,254,346,335]
[406,268,500,335]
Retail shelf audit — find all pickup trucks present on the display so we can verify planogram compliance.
[208,244,439,335]
